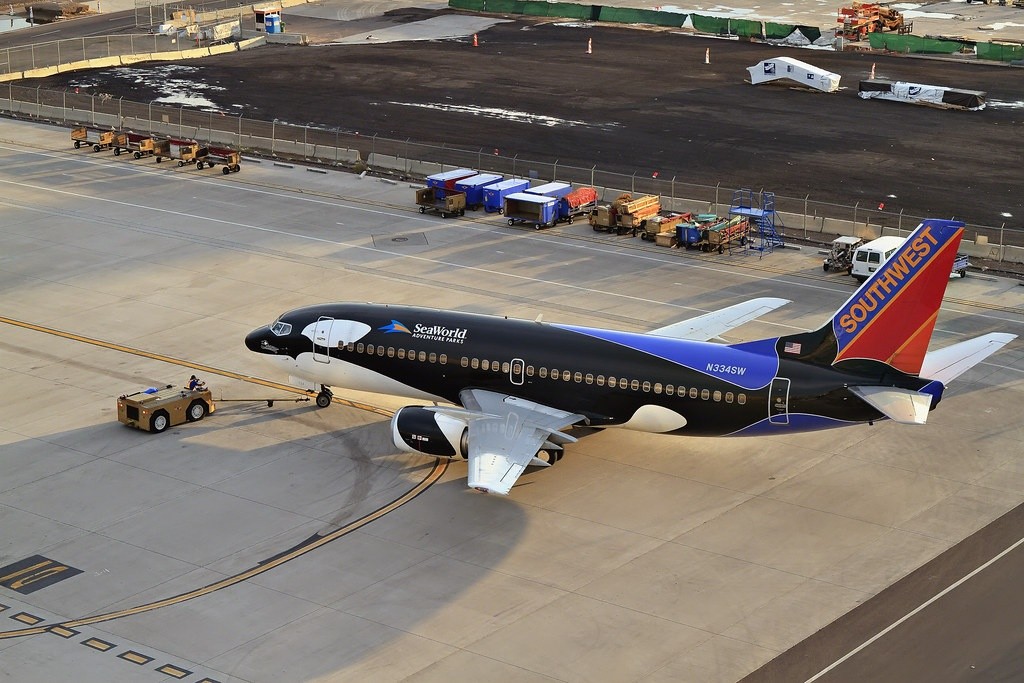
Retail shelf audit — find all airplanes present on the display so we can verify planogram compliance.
[242,213,1022,498]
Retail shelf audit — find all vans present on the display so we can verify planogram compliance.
[848,236,907,285]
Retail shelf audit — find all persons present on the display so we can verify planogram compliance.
[186,375,206,391]
